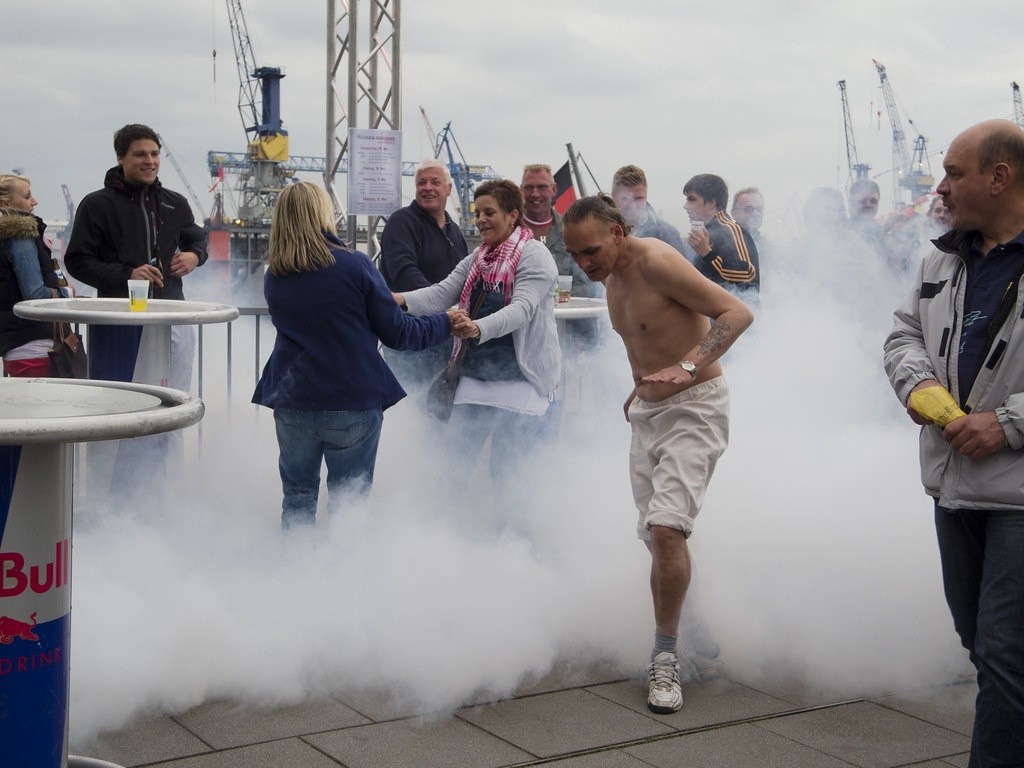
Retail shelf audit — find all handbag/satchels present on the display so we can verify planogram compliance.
[426,367,460,424]
[46,288,87,378]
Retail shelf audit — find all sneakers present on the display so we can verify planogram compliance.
[645,652,683,714]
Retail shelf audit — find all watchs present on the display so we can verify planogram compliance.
[677,361,699,381]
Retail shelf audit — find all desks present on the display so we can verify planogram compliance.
[12,299,240,509]
[554,296,612,433]
[0,378,207,768]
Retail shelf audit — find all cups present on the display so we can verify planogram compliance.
[557,275,573,302]
[127,280,149,312]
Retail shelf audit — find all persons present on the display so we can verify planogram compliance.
[383,164,945,494]
[884,120,1024,768]
[64,123,209,382]
[562,192,754,714]
[251,182,468,529]
[0,175,77,378]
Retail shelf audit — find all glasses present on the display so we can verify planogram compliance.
[522,185,552,191]
[888,230,914,240]
[857,198,878,205]
[734,206,765,214]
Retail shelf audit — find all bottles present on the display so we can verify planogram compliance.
[551,254,559,305]
[51,257,68,287]
